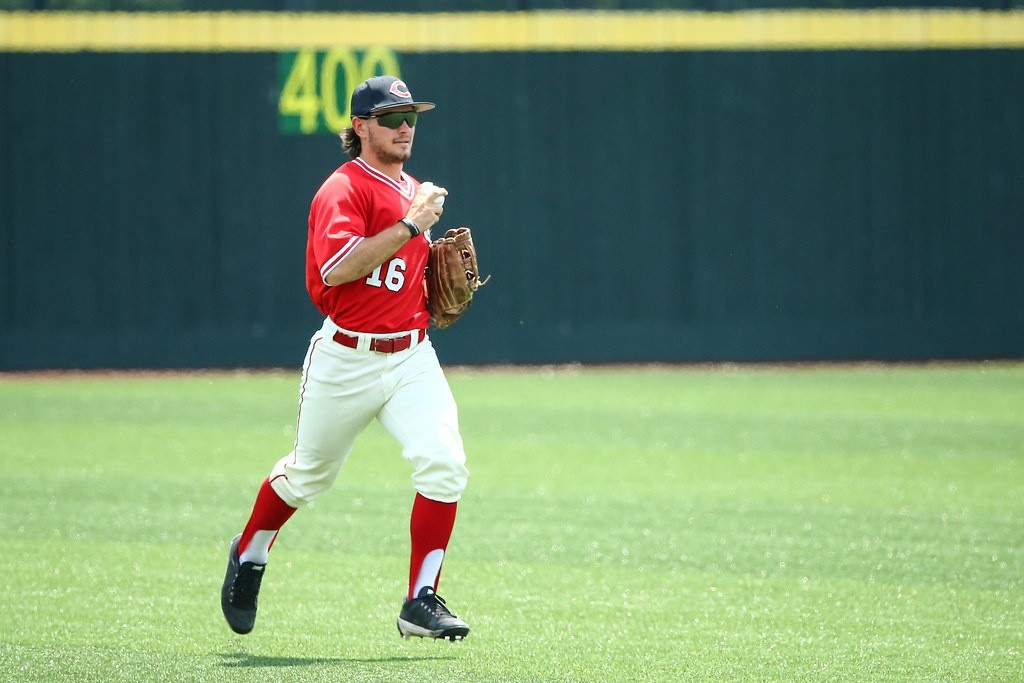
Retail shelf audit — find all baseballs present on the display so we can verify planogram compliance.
[422,183,444,205]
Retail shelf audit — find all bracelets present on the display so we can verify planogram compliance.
[399,216,420,238]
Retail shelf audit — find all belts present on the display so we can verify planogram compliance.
[333,329,425,354]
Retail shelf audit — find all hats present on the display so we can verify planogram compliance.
[350,75,436,112]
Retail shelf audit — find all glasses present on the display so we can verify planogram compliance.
[350,110,417,129]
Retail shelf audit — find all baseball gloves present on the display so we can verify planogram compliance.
[423,227,480,330]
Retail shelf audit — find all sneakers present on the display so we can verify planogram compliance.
[221,534,267,634]
[397,585,470,642]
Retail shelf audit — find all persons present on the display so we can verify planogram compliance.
[221,76,471,641]
